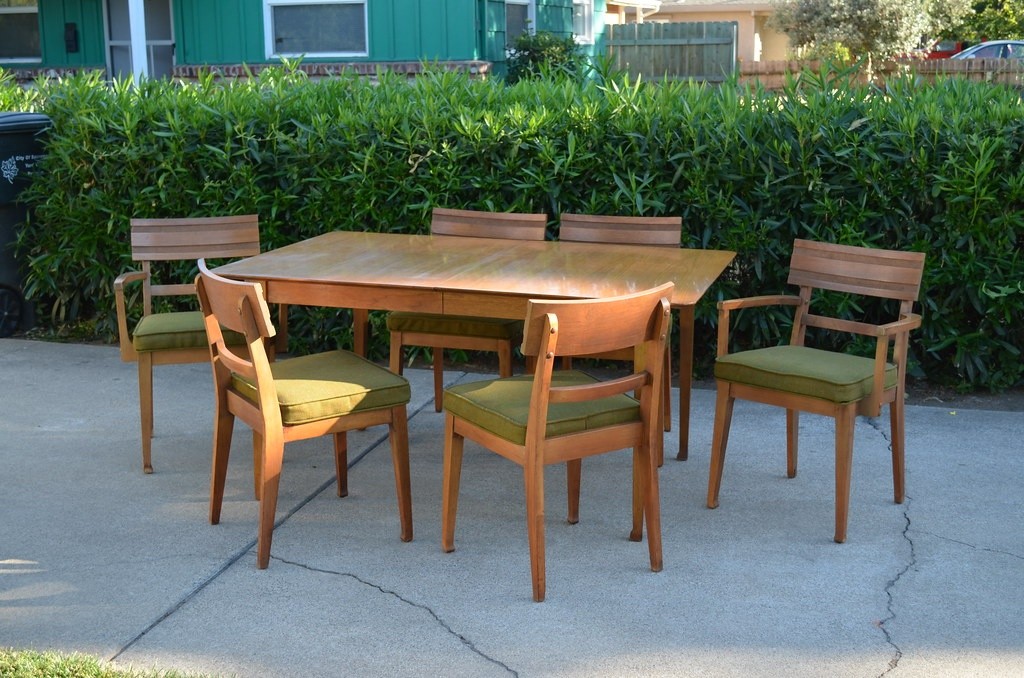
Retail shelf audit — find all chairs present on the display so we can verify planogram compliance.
[442,281,675,602]
[194,258,413,569]
[386,207,547,444]
[707,239,926,542]
[526,212,682,468]
[114,214,288,473]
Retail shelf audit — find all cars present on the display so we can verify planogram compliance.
[0,113,57,338]
[951,39,1024,59]
[889,37,1011,59]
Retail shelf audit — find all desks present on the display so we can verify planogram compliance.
[209,231,736,542]
